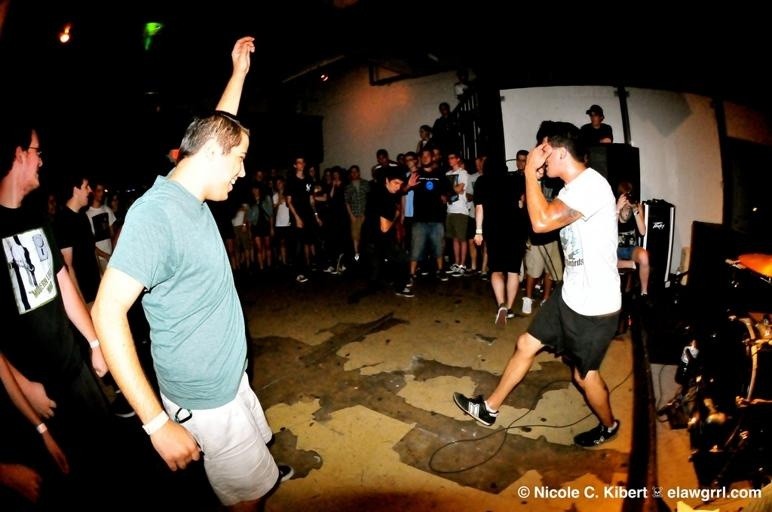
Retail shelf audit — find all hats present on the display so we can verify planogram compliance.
[586,105,603,115]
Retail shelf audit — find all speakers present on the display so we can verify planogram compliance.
[589,142,640,202]
[642,199,676,293]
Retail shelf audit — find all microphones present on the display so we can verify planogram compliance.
[517,164,546,209]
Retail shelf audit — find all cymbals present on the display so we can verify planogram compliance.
[727,254,770,278]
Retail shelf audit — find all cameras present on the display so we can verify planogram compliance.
[625,192,638,204]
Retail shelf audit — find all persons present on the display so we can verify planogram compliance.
[1,32,772,512]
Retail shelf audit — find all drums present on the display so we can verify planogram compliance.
[685,313,771,475]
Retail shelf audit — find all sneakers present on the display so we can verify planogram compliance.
[453,392,499,426]
[276,465,294,482]
[495,297,533,327]
[396,276,415,298]
[435,264,489,281]
[296,253,347,282]
[574,420,619,447]
